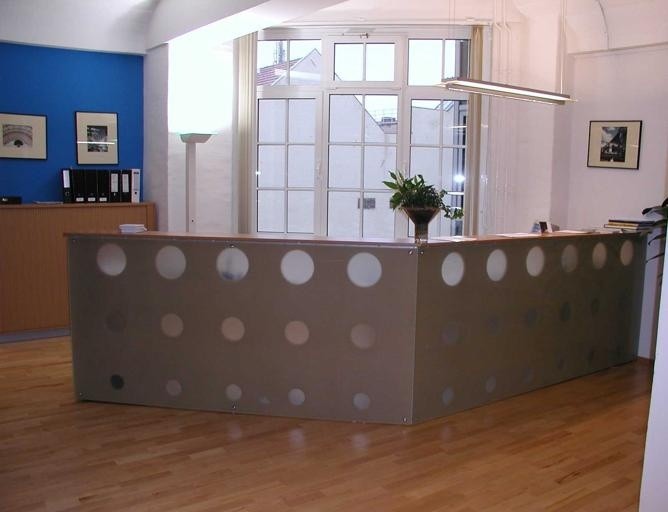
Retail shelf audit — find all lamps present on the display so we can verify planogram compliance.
[442,0,579,107]
[181,132,212,144]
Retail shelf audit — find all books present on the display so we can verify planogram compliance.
[604,219,655,231]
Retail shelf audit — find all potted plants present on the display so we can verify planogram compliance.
[383,170,465,239]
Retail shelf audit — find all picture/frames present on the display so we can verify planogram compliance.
[587,120,642,170]
[74,110,119,165]
[0,111,48,161]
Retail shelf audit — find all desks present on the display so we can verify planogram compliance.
[0,202,156,335]
